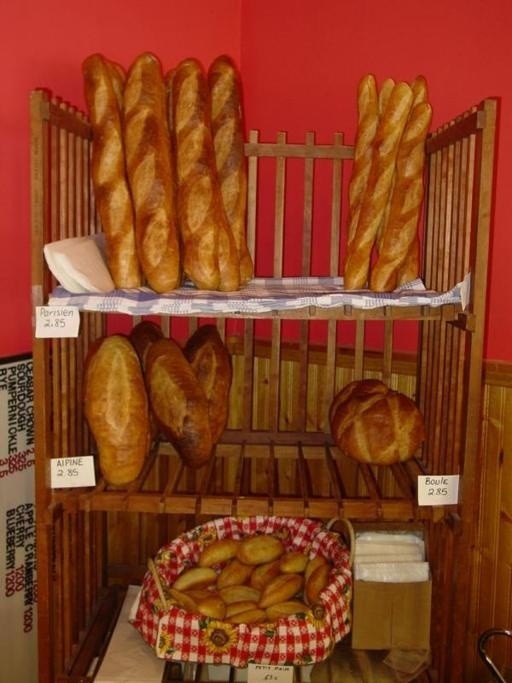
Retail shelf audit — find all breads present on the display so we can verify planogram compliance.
[344,73,432,293]
[87,319,233,485]
[83,52,256,291]
[328,376,426,465]
[169,534,332,624]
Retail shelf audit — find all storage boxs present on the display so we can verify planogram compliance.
[351,575,432,653]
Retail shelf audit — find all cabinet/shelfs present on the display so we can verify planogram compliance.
[27,84,501,683]
